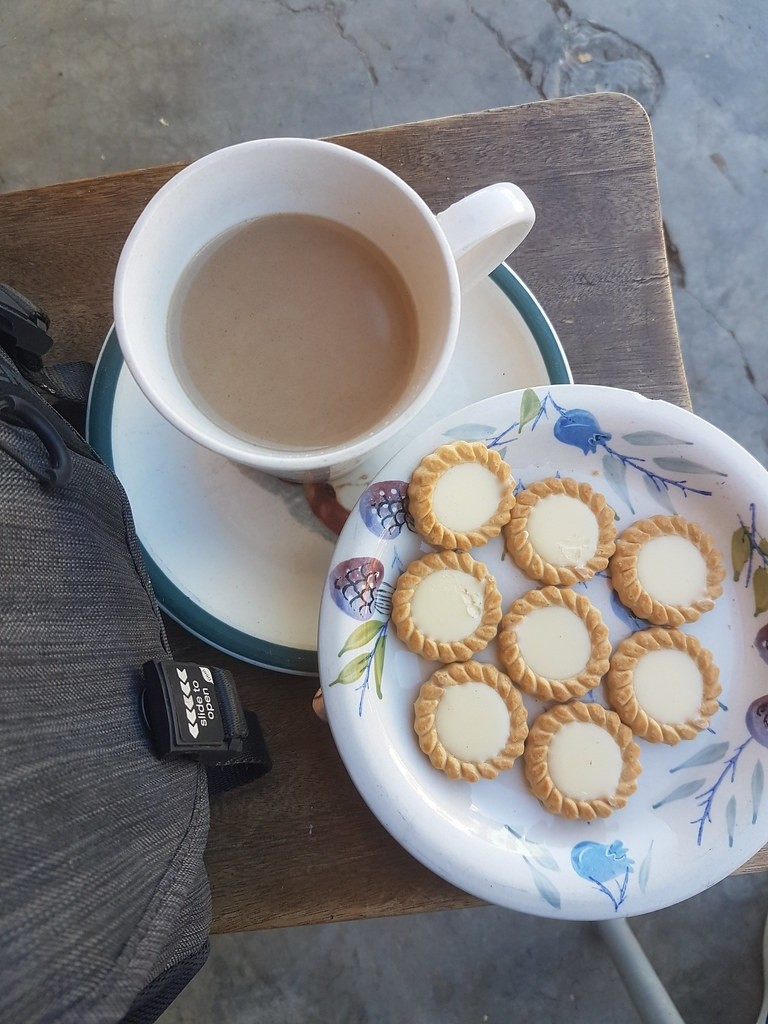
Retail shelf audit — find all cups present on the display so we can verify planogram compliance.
[112,136,538,487]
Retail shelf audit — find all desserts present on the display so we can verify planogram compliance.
[390,440,725,821]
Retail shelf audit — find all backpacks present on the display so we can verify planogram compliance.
[0,283,271,1022]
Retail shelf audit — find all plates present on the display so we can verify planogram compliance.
[316,383,768,923]
[83,263,574,679]
